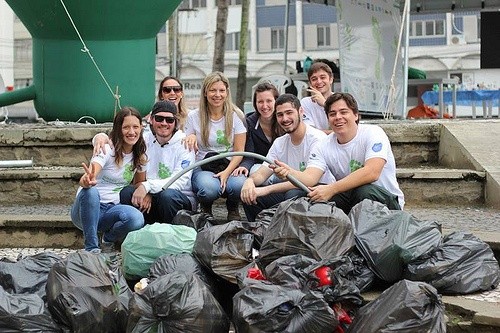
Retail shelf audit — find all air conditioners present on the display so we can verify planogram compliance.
[451,34,466,45]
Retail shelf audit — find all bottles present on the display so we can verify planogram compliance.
[302,56,312,72]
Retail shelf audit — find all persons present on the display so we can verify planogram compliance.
[147,76,188,133]
[268,91,406,215]
[91,100,196,225]
[299,62,336,135]
[240,93,337,223]
[70,108,152,252]
[181,72,248,221]
[231,82,287,185]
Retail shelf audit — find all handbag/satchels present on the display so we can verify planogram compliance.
[201,152,230,173]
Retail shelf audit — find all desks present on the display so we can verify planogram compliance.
[420,88,500,119]
[408,78,459,119]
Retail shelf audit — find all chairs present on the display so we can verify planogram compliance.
[293,80,309,100]
[252,75,291,106]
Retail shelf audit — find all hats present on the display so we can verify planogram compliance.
[151,102,177,116]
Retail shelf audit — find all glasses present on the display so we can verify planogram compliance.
[163,86,182,94]
[155,115,175,123]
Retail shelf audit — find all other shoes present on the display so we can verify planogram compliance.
[227,209,241,221]
[100,233,115,254]
[201,209,212,214]
